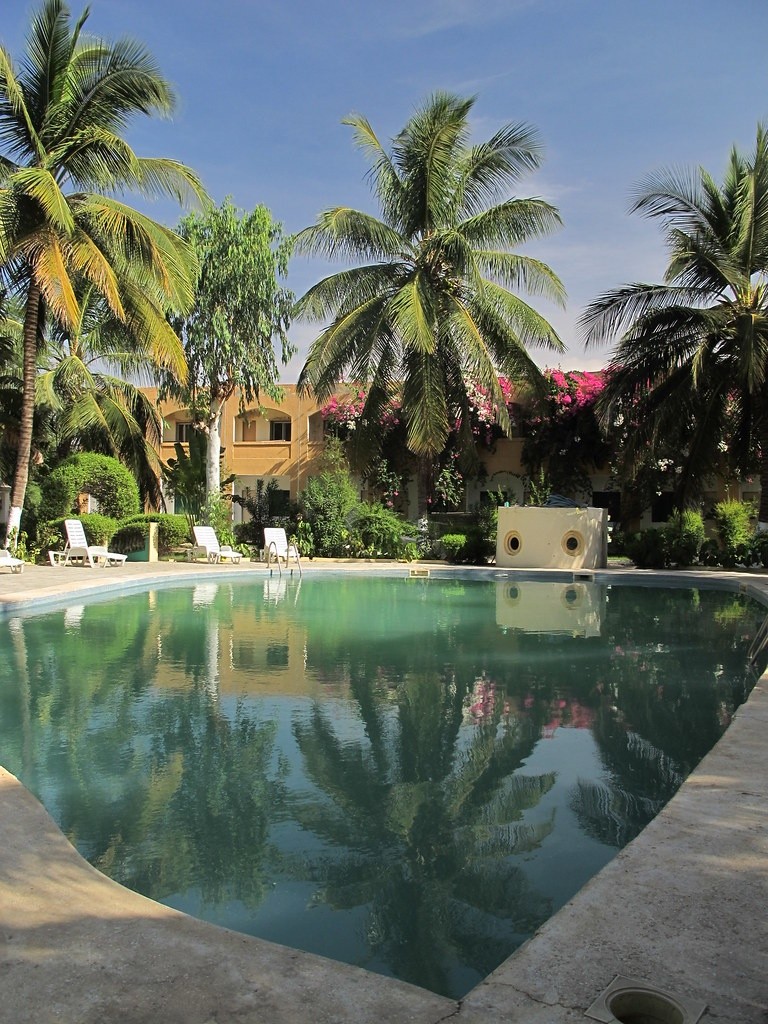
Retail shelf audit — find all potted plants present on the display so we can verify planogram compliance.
[440,534,466,564]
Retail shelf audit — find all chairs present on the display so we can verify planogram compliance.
[0,550,24,573]
[260,528,300,563]
[191,525,242,565]
[48,520,128,569]
